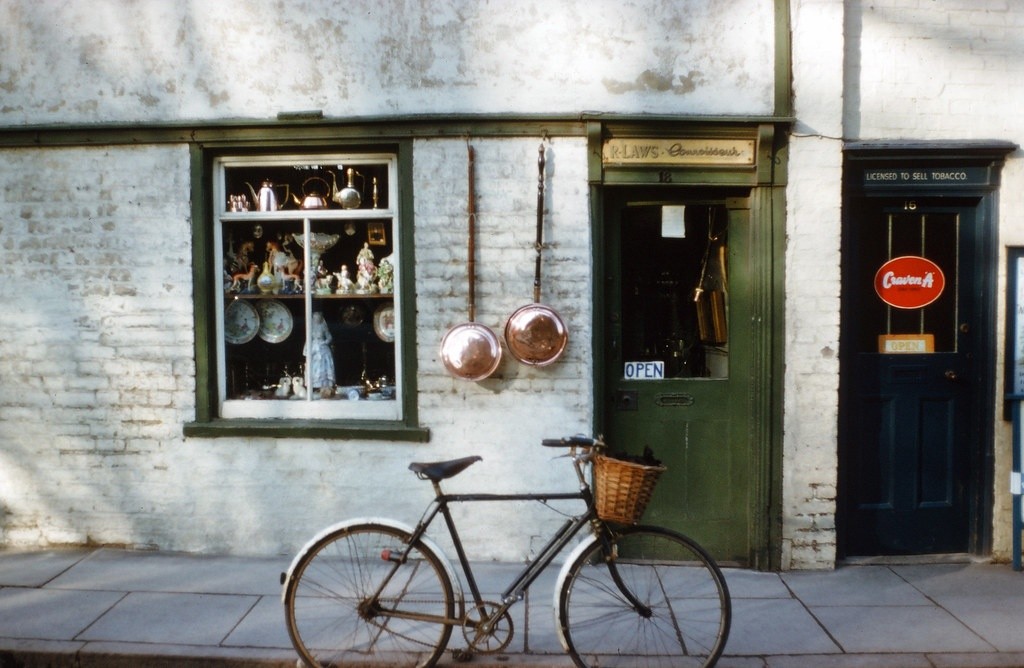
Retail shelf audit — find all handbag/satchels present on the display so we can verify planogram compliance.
[694,290,727,342]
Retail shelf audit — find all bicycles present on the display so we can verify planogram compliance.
[278,437,732,668]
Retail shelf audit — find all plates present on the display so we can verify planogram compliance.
[373,301,394,343]
[254,299,293,344]
[224,300,260,345]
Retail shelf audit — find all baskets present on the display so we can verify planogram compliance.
[592,452,667,526]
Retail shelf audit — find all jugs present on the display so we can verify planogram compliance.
[290,177,330,210]
[243,176,289,211]
[326,168,365,209]
